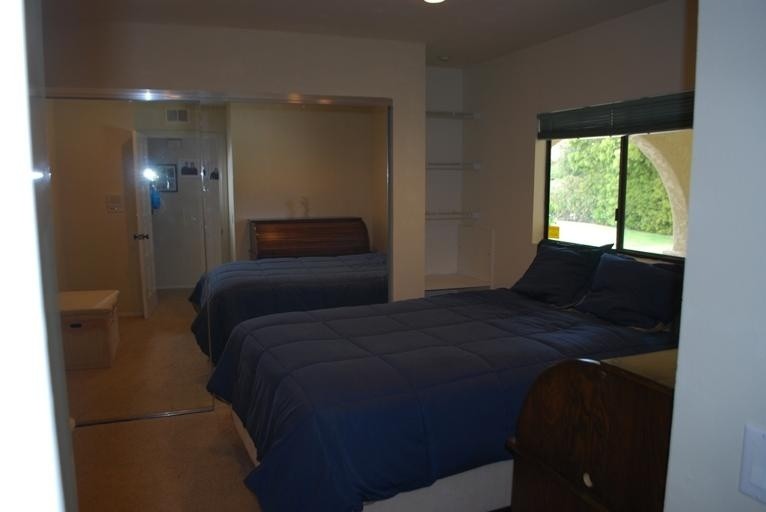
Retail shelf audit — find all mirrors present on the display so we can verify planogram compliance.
[50,95,397,428]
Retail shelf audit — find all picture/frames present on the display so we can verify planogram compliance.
[151,163,179,193]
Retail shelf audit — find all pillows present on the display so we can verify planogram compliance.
[507,236,614,306]
[573,252,681,326]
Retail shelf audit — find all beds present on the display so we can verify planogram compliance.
[179,252,387,378]
[207,236,684,512]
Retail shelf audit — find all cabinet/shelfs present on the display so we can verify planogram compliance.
[246,214,371,258]
[503,346,678,512]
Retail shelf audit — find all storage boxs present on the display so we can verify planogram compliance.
[56,286,124,372]
